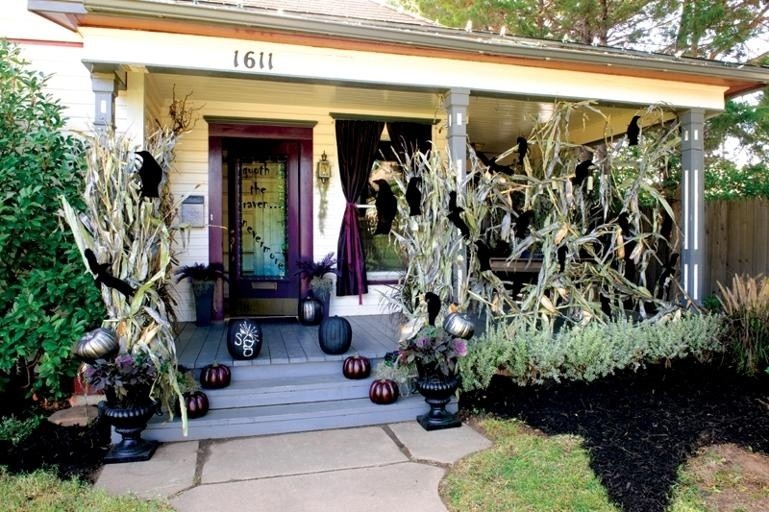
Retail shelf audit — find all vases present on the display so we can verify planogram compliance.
[85,354,157,401]
[393,324,468,377]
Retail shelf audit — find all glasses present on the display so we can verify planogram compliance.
[317,149,332,184]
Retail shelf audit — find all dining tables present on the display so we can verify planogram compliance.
[174,261,229,327]
[290,252,344,319]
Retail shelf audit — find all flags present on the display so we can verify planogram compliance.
[98,385,160,463]
[415,358,461,432]
